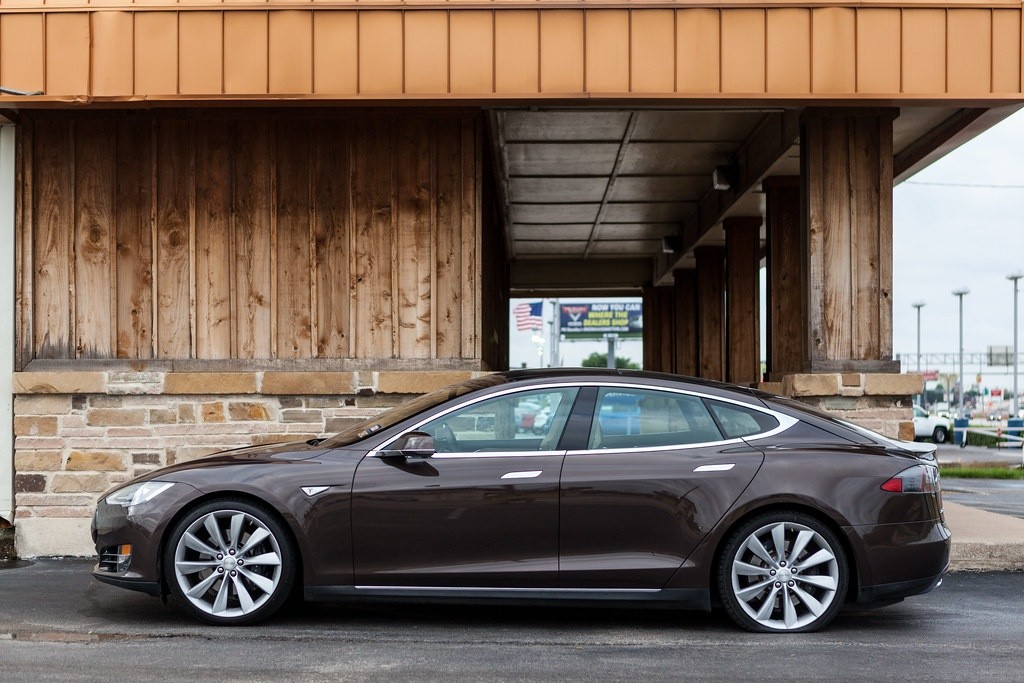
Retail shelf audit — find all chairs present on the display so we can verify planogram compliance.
[594,421,604,450]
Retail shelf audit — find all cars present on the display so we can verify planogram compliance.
[90,365,953,634]
[913,404,952,444]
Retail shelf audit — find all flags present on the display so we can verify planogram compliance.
[513,301,543,330]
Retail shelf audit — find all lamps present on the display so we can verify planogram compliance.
[712,165,734,190]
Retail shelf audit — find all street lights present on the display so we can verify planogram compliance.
[912,302,926,374]
[1005,273,1024,417]
[952,288,970,415]
